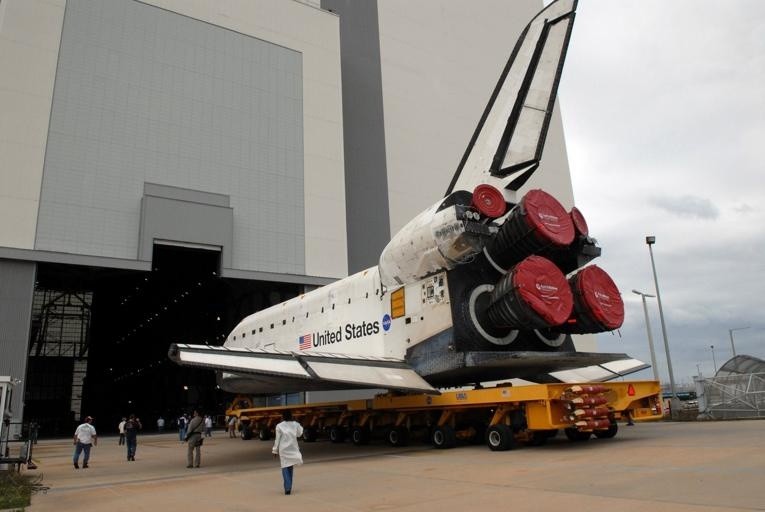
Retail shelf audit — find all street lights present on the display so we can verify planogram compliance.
[645,236,677,394]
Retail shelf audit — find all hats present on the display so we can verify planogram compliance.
[86,416,93,423]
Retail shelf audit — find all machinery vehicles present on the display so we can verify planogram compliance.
[224,380,663,451]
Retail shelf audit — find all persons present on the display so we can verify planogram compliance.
[156,416,165,434]
[271,409,303,495]
[123,414,139,461]
[118,417,127,446]
[177,406,212,468]
[227,415,236,438]
[29,417,40,444]
[72,415,98,469]
